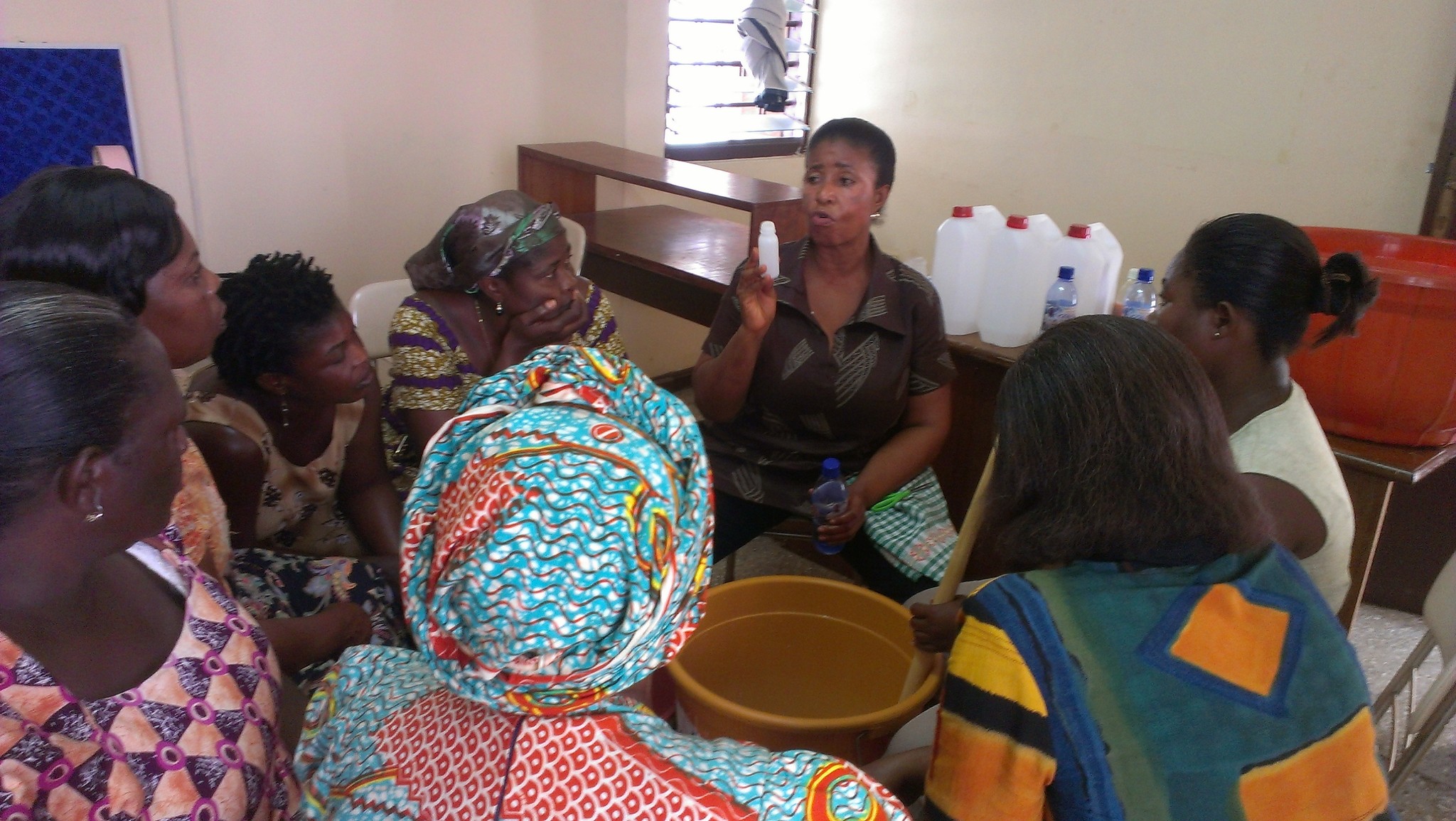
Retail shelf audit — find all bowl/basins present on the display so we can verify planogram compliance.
[1284,226,1456,447]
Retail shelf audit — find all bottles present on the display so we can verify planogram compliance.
[759,221,779,280]
[980,214,1064,348]
[1124,268,1157,319]
[935,205,1009,335]
[1048,223,1123,318]
[1112,268,1139,317]
[813,458,849,555]
[1041,266,1077,336]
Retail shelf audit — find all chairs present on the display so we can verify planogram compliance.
[1369,551,1456,797]
[343,278,417,387]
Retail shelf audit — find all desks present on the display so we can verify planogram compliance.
[932,329,1456,640]
[519,140,810,328]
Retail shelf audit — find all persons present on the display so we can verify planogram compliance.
[908,213,1381,653]
[290,345,913,821]
[379,189,629,511]
[907,314,1400,821]
[691,117,959,605]
[0,164,415,821]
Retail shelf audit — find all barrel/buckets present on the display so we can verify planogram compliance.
[665,575,945,768]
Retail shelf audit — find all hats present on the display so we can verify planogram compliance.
[399,344,715,715]
